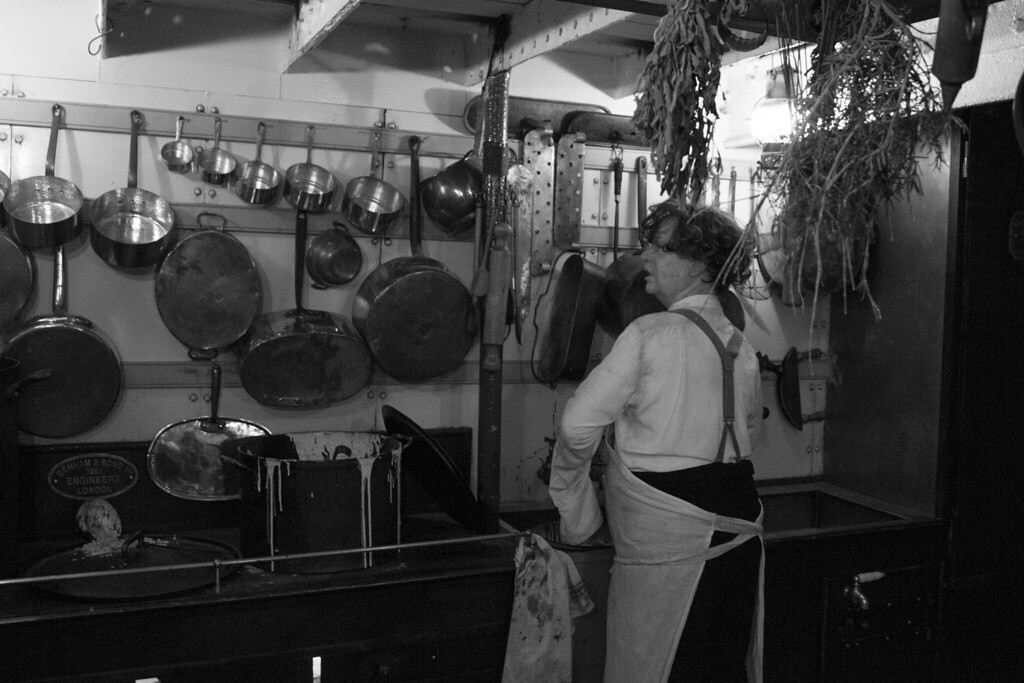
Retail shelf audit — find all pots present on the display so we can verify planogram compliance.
[349,136,480,384]
[160,114,194,172]
[283,126,338,213]
[90,111,174,269]
[2,103,84,246]
[234,123,280,204]
[0,156,665,578]
[341,135,404,234]
[198,118,237,184]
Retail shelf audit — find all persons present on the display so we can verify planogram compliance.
[549,198,765,683]
